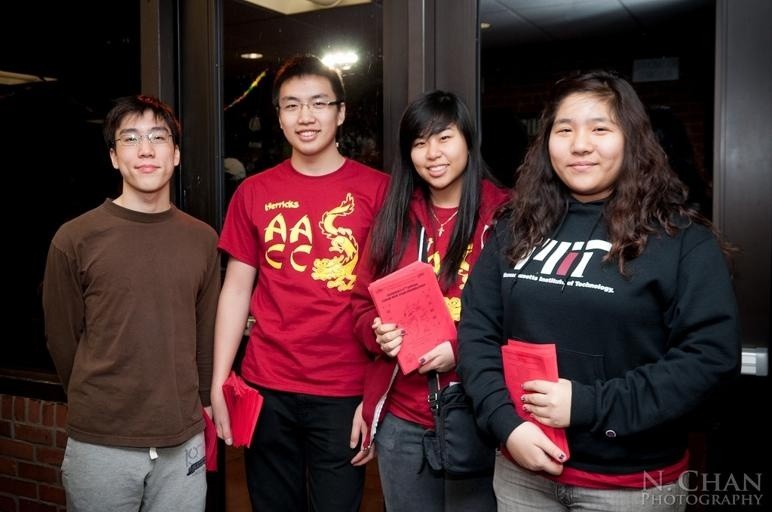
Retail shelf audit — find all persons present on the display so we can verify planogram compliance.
[211,55,400,512]
[43,94,221,512]
[348,88,517,512]
[456,69,742,511]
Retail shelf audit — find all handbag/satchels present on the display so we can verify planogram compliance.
[416,218,493,483]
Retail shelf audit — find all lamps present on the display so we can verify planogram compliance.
[317,31,358,68]
[238,26,264,60]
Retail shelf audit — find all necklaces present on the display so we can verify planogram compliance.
[427,207,460,237]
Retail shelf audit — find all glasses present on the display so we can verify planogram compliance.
[275,99,340,112]
[114,132,175,145]
